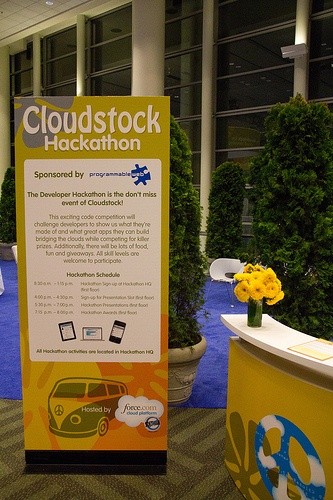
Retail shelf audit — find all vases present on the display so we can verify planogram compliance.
[247,297,263,329]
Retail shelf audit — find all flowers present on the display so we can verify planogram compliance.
[232,263,285,306]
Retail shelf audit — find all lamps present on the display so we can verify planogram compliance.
[280,42,307,60]
